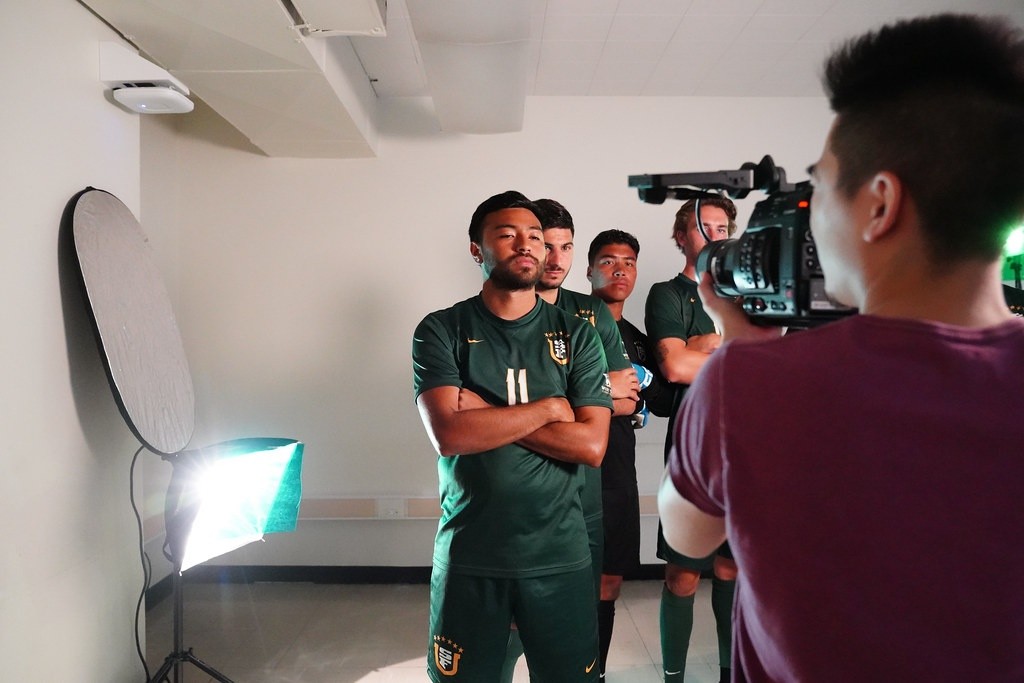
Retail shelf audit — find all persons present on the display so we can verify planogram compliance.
[587,229,646,683]
[643,197,736,683]
[412,190,615,683]
[657,15,1023,683]
[503,199,641,683]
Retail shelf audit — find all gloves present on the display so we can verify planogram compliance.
[631,362,654,390]
[631,399,650,430]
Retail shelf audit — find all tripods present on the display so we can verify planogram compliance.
[151,557,234,683]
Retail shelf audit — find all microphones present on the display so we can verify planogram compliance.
[638,186,720,205]
